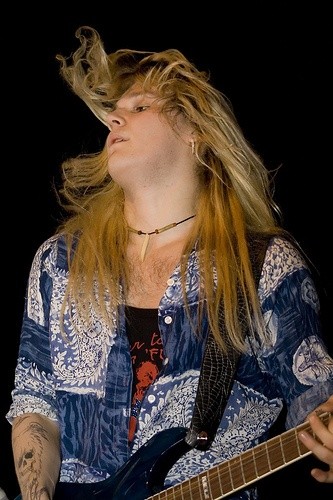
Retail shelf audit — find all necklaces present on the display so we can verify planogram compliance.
[127,213,199,236]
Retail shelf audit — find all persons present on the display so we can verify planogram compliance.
[7,49,332,500]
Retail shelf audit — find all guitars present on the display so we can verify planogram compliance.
[13,397,332,500]
